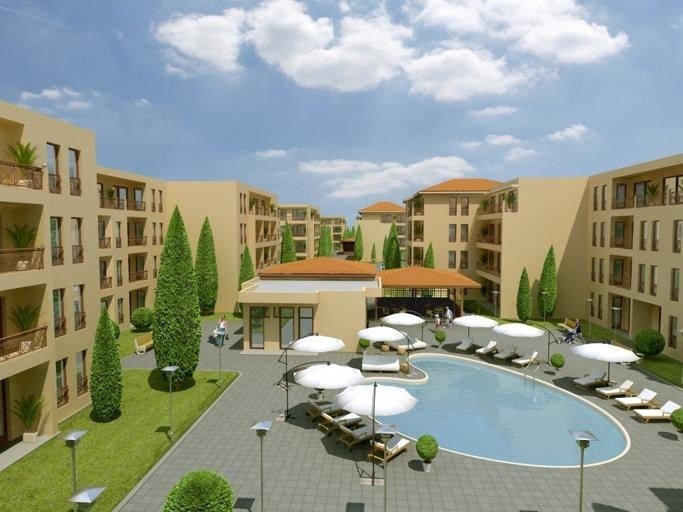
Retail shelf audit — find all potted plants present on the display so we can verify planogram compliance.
[671,408,683,440]
[7,139,40,187]
[8,304,43,353]
[550,352,567,376]
[640,184,662,206]
[11,395,44,442]
[434,330,447,349]
[5,224,37,271]
[359,339,370,353]
[415,434,439,473]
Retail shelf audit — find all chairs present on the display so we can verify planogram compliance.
[596,380,634,400]
[574,368,605,394]
[475,340,497,357]
[361,345,400,372]
[633,400,682,424]
[615,388,656,412]
[455,338,473,351]
[512,351,537,368]
[384,339,427,350]
[367,435,411,467]
[338,424,373,451]
[320,412,363,435]
[493,344,519,362]
[306,399,337,423]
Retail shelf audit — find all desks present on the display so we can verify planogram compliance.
[374,423,396,441]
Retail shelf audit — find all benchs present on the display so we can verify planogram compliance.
[134,331,155,354]
[557,318,576,334]
[610,341,630,363]
